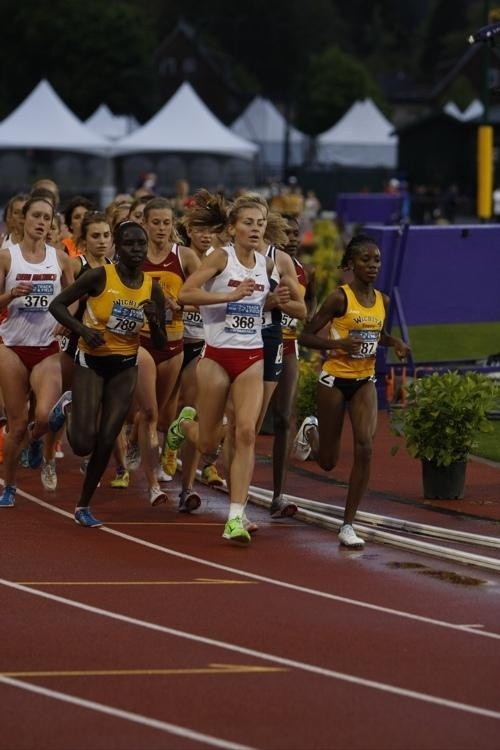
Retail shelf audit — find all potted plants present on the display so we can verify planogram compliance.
[387,370,499,499]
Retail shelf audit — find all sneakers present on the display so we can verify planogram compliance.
[122,443,141,472]
[160,434,182,477]
[149,485,168,506]
[289,415,319,465]
[0,485,17,507]
[26,421,66,492]
[48,390,72,434]
[73,507,104,528]
[177,491,202,512]
[336,523,367,548]
[165,405,197,451]
[221,511,261,546]
[269,494,298,520]
[109,468,130,487]
[199,463,225,488]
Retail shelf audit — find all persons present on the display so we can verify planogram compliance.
[3,171,477,556]
